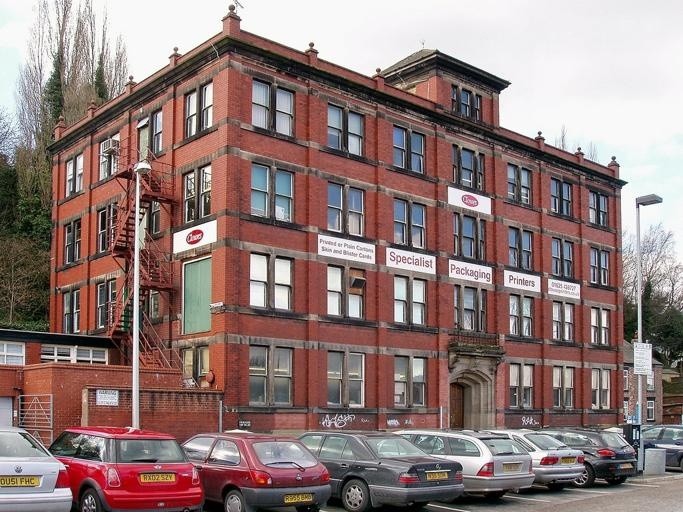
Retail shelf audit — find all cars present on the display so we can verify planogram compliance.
[0,425,72,512]
[478,429,585,493]
[47,423,204,511]
[534,428,637,487]
[295,430,463,512]
[392,429,536,503]
[179,431,331,512]
[639,424,683,472]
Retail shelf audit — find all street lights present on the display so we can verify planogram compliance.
[130,161,153,429]
[634,193,662,476]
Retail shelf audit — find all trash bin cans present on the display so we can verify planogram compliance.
[621,425,640,452]
